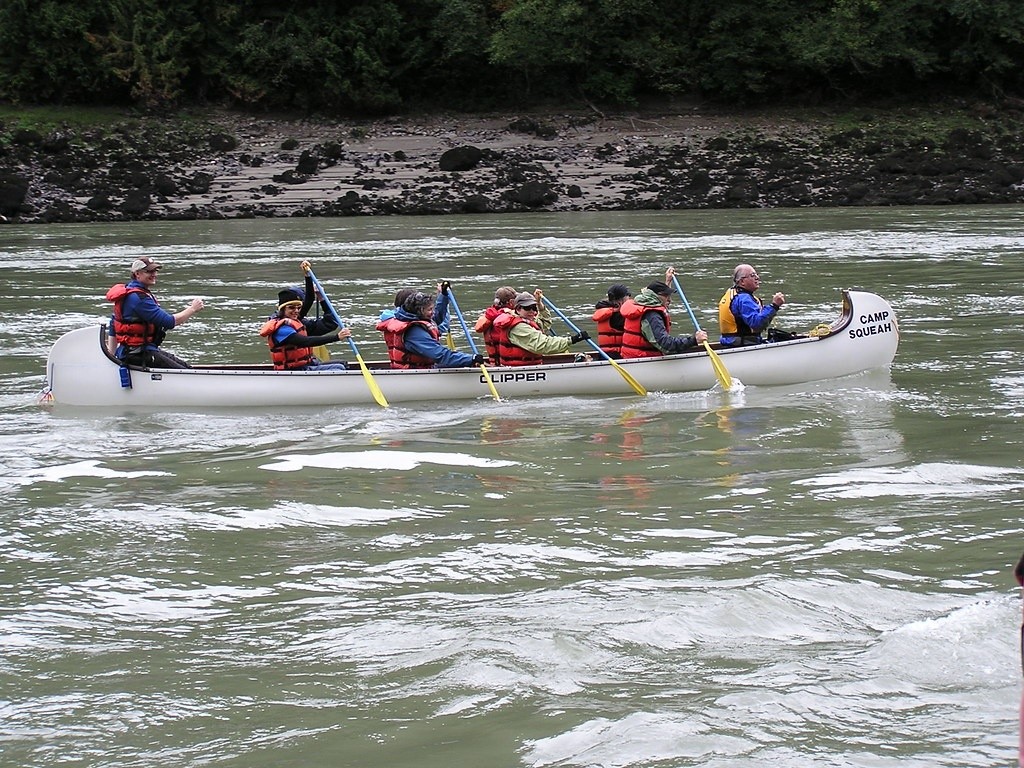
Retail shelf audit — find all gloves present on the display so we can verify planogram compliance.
[571,331,591,345]
[472,353,484,364]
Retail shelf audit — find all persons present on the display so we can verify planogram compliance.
[260,285,351,371]
[476,287,518,366]
[107,257,203,370]
[388,280,484,371]
[593,285,632,362]
[376,290,416,360]
[496,289,591,368]
[719,264,786,349]
[620,265,708,359]
[271,261,315,322]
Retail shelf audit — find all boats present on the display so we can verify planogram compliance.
[31,288,899,408]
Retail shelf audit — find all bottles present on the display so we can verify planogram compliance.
[119,364,129,387]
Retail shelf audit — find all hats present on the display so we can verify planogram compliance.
[648,281,678,295]
[495,286,520,304]
[608,284,628,299]
[132,256,162,274]
[278,289,305,311]
[513,291,540,310]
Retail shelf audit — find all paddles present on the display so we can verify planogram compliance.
[444,280,501,403]
[303,263,390,408]
[445,328,456,351]
[549,327,569,353]
[539,293,647,397]
[313,291,330,362]
[670,267,732,389]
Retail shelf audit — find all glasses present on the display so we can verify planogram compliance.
[414,292,423,301]
[743,272,758,278]
[520,304,536,311]
[628,293,631,297]
